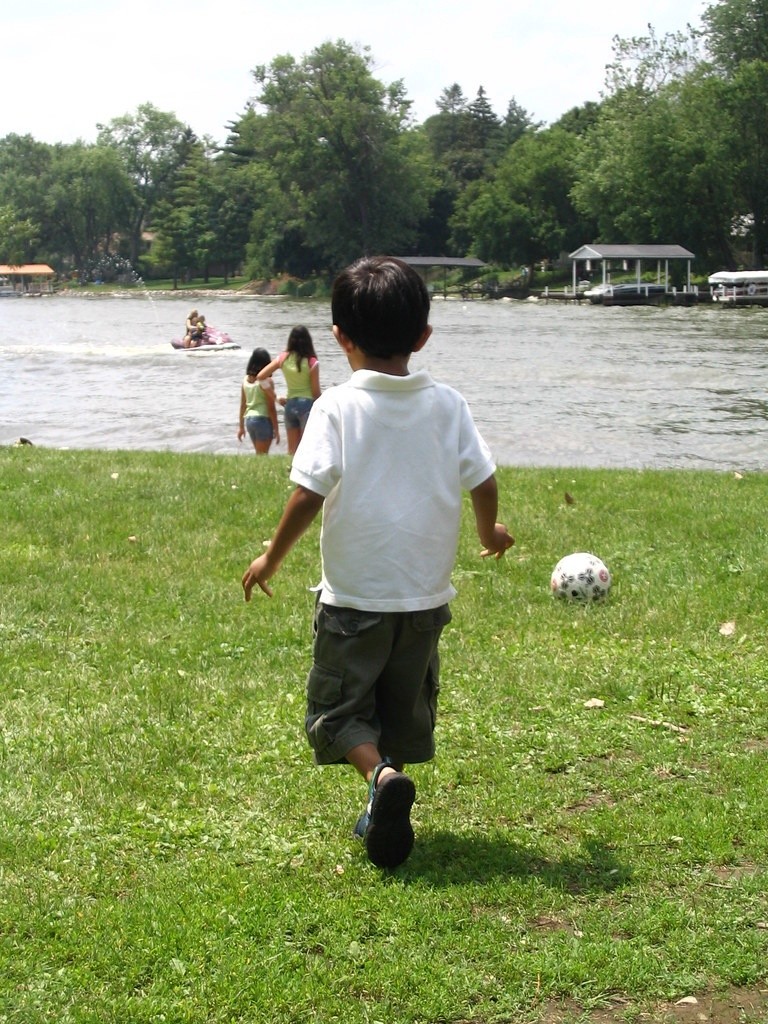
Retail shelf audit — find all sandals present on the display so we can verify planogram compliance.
[354,762,415,866]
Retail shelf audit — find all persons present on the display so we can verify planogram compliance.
[237,348,281,455]
[240,256,516,871]
[256,326,321,455]
[183,309,206,348]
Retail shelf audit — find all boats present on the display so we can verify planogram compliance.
[584,281,667,305]
[170,325,243,353]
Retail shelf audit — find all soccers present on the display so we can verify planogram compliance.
[551,552,612,604]
